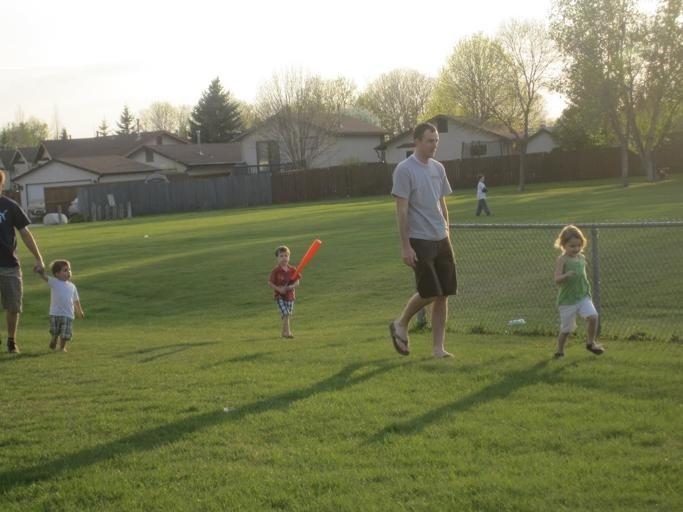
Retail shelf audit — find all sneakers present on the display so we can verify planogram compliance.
[7,337,18,353]
[587,343,604,354]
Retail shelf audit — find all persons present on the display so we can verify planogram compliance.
[30,257,84,353]
[474,171,493,221]
[0,168,44,354]
[265,246,302,340]
[551,223,605,358]
[386,123,457,359]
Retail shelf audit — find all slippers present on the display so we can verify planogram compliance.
[390,323,410,355]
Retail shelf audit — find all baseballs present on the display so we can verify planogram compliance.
[222,407,229,412]
[144,235,147,237]
